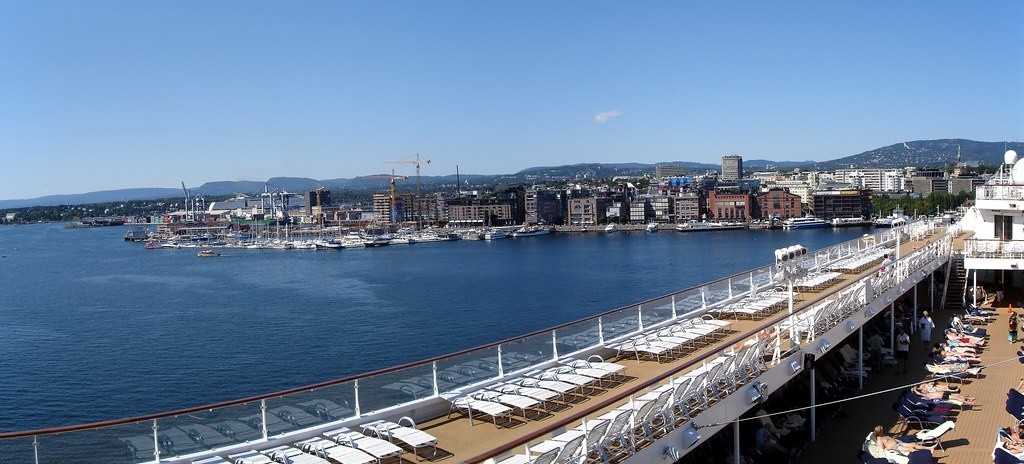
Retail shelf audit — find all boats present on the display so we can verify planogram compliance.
[677,219,745,232]
[581,226,587,232]
[605,222,620,233]
[766,215,872,230]
[646,221,659,233]
[197,250,222,258]
[123,221,552,249]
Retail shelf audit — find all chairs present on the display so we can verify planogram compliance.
[969,241,1024,259]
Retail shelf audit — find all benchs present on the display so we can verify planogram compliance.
[992,387,1024,464]
[861,419,955,464]
[359,416,439,461]
[380,345,552,401]
[1016,302,1024,364]
[117,393,357,461]
[707,285,801,322]
[464,356,629,422]
[604,314,735,365]
[439,388,514,429]
[796,244,861,269]
[482,327,783,464]
[787,268,845,294]
[191,445,332,464]
[730,270,777,291]
[544,311,668,349]
[894,384,965,430]
[923,301,996,385]
[323,426,405,464]
[821,246,896,275]
[654,286,745,314]
[760,234,954,343]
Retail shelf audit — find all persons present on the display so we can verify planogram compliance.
[913,382,976,406]
[722,408,807,464]
[868,327,885,377]
[876,251,895,278]
[883,306,914,351]
[924,301,988,372]
[717,326,775,356]
[874,425,939,455]
[894,326,911,375]
[1003,424,1024,454]
[918,310,936,349]
[1008,311,1020,344]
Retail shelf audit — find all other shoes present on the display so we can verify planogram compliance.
[894,372,900,375]
[902,370,907,375]
[926,346,929,349]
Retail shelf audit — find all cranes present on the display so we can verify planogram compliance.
[362,168,408,226]
[383,152,431,200]
